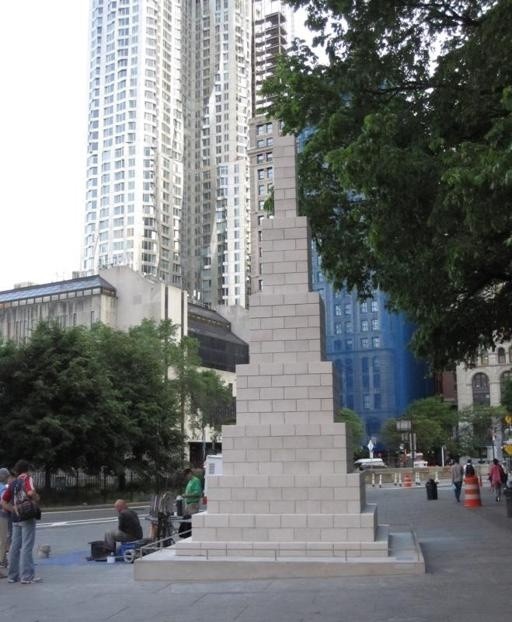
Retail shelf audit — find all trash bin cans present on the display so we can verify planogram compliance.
[426,479,436,499]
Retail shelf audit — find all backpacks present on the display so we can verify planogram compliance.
[179,514,192,538]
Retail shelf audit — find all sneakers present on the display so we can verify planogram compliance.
[8,577,42,585]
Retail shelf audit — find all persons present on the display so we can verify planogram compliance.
[489,459,505,501]
[180,469,202,516]
[0,458,41,584]
[451,458,477,502]
[105,499,143,550]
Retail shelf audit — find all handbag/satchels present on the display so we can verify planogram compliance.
[11,499,41,522]
